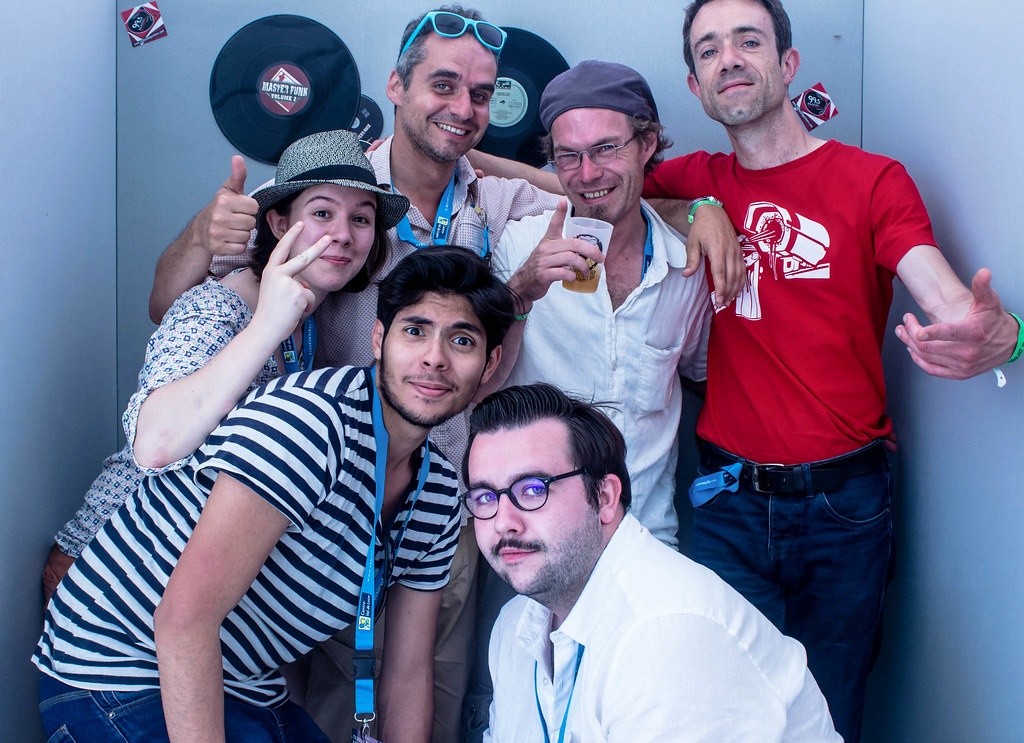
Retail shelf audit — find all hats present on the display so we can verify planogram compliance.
[251,129,410,232]
[539,60,660,132]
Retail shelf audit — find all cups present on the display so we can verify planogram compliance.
[561,215,614,294]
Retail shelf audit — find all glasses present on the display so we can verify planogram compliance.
[397,11,508,62]
[457,467,585,520]
[547,132,638,171]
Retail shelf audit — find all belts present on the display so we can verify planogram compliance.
[698,440,886,496]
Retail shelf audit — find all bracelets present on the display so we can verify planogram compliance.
[992,312,1024,388]
[686,196,723,224]
[514,312,529,321]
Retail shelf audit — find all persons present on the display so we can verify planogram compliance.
[461,383,845,743]
[146,4,745,743]
[461,58,708,743]
[43,128,411,604]
[31,243,516,743]
[365,1,1024,742]
